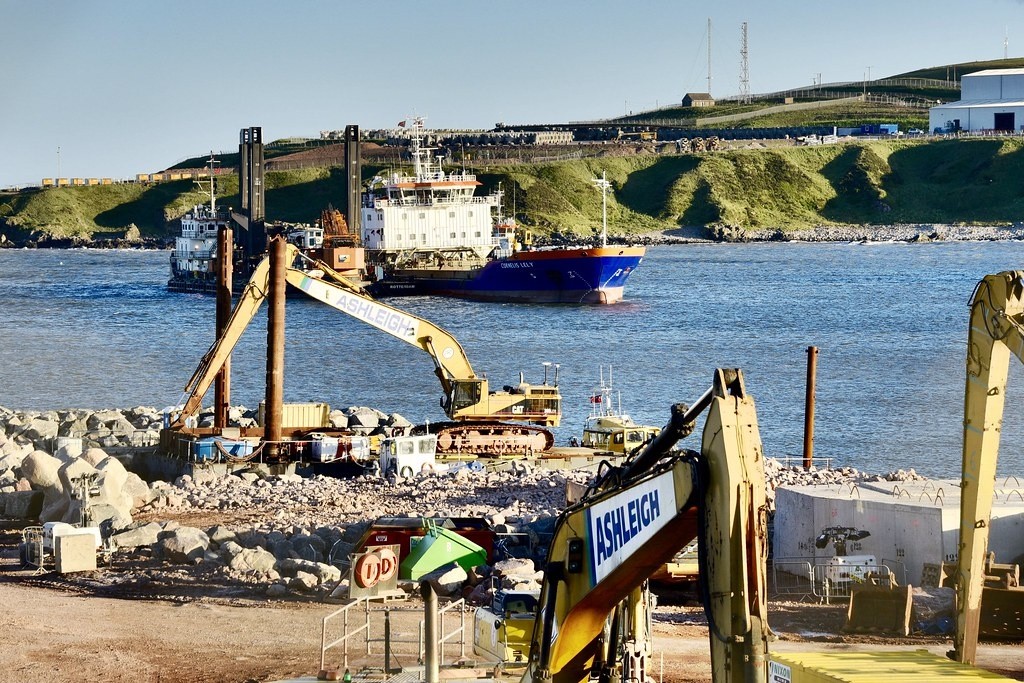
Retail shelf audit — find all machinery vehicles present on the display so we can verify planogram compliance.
[472,365,779,682]
[945,268,1024,667]
[153,245,562,455]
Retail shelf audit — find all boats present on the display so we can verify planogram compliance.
[166,115,647,306]
[571,363,680,455]
